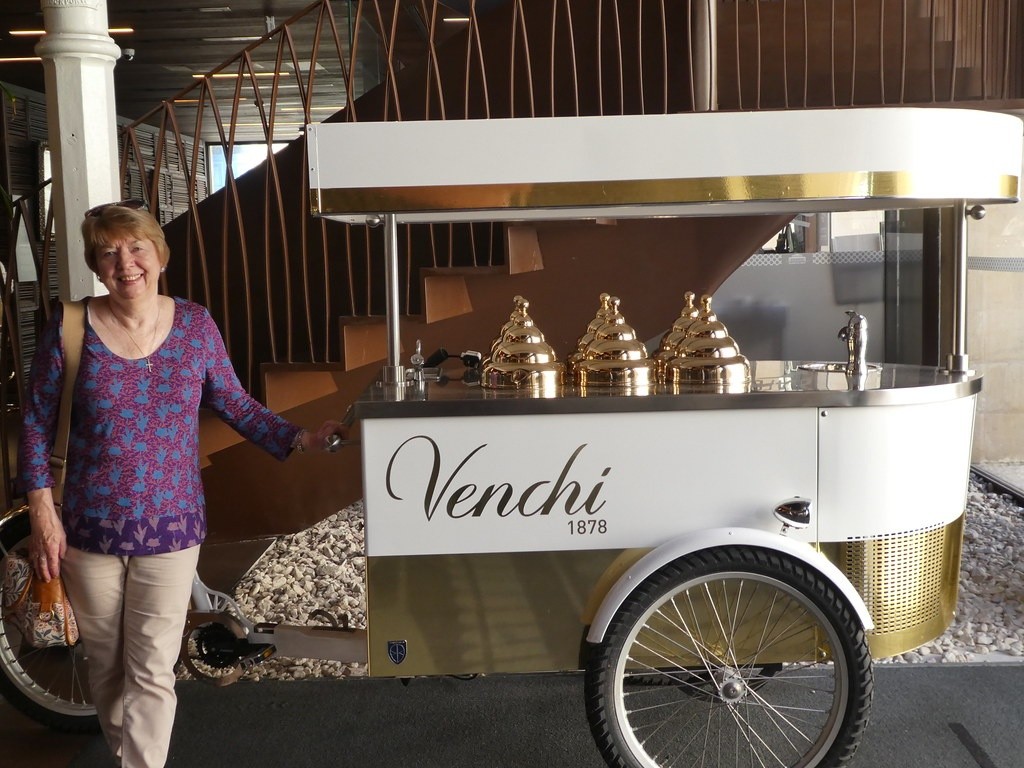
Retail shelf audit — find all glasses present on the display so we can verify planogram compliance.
[84,198,152,219]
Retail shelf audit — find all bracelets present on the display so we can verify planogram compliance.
[297,427,306,454]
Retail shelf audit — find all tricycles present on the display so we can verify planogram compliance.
[0,105,1024,768]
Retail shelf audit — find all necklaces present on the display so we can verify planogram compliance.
[105,295,162,373]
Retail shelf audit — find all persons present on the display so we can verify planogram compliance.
[17,202,353,768]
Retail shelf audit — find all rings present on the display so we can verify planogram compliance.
[38,556,47,561]
[32,552,38,558]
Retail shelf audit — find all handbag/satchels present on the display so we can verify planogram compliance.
[0,495,80,649]
